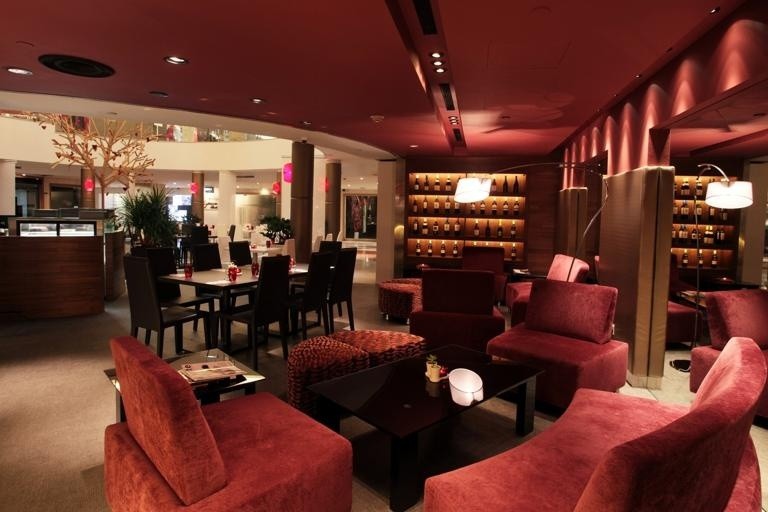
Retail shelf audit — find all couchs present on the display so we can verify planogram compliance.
[498,252,594,328]
[688,289,768,421]
[408,267,504,345]
[289,334,368,411]
[483,281,631,421]
[376,277,424,323]
[668,302,702,348]
[99,331,356,512]
[331,324,426,364]
[460,244,506,274]
[424,336,768,512]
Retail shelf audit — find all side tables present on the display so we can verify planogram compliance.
[98,343,272,425]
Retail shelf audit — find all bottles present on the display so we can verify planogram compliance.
[673,201,678,215]
[413,218,418,235]
[454,219,461,236]
[414,178,419,190]
[715,226,720,240]
[454,202,460,214]
[432,219,439,236]
[424,176,429,190]
[480,201,485,214]
[711,249,718,268]
[720,226,724,240]
[709,225,714,245]
[470,202,475,215]
[441,241,446,255]
[512,243,517,258]
[680,178,690,197]
[502,199,509,215]
[415,240,420,255]
[680,201,689,216]
[510,221,516,239]
[492,198,497,214]
[497,220,503,238]
[672,226,675,239]
[503,176,508,193]
[698,250,703,267]
[434,176,440,190]
[474,221,479,239]
[709,206,714,217]
[679,225,688,242]
[514,199,519,215]
[445,196,450,214]
[704,225,709,244]
[681,249,689,266]
[413,198,418,214]
[719,209,728,220]
[423,197,428,214]
[513,176,520,193]
[422,219,429,235]
[696,202,702,216]
[435,196,440,214]
[691,226,700,241]
[693,180,702,197]
[251,252,259,276]
[428,240,432,256]
[486,219,490,239]
[444,219,450,236]
[453,241,458,256]
[491,178,497,194]
[674,181,677,197]
[446,177,451,190]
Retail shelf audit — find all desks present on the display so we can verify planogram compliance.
[675,290,712,313]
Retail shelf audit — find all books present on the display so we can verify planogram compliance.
[178,361,248,391]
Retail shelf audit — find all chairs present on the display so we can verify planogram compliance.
[188,239,252,331]
[319,240,343,252]
[228,223,236,241]
[220,255,290,369]
[180,225,210,267]
[122,255,212,358]
[293,246,359,332]
[289,248,332,339]
[130,245,216,354]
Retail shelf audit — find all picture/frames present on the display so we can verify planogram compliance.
[342,195,376,243]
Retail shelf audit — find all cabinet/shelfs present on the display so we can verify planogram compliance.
[675,177,740,286]
[403,173,525,277]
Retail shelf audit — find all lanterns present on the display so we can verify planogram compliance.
[84,178,94,191]
[190,182,198,194]
[272,181,281,194]
[283,162,293,183]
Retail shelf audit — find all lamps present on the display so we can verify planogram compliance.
[668,162,754,372]
[453,161,611,284]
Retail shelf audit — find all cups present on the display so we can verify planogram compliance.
[227,264,237,281]
[183,262,192,278]
[289,257,294,270]
